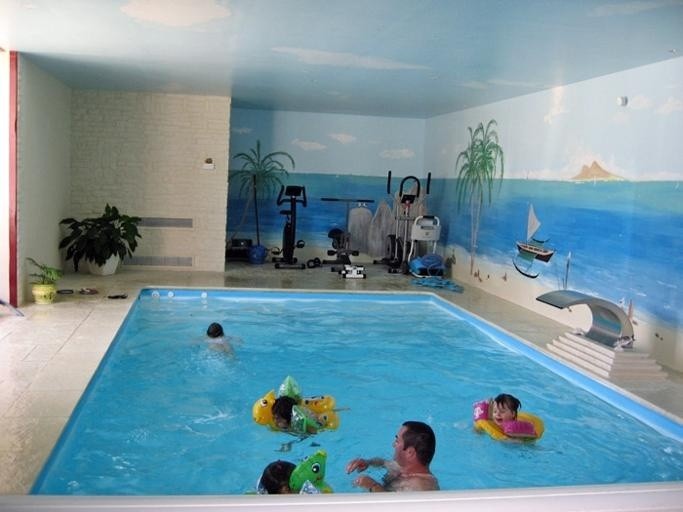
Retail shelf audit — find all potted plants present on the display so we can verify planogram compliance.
[56,201,144,277]
[24,255,65,306]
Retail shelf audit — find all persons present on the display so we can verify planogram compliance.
[270,396,298,430]
[347,421,440,493]
[262,460,296,494]
[491,394,522,428]
[205,322,234,356]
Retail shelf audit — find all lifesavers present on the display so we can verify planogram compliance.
[252,375,339,432]
[246,450,335,495]
[473,398,545,444]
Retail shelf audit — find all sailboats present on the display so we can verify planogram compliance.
[512,200,557,266]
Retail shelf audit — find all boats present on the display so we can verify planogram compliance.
[512,250,540,279]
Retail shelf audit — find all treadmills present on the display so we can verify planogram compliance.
[409,213,445,280]
